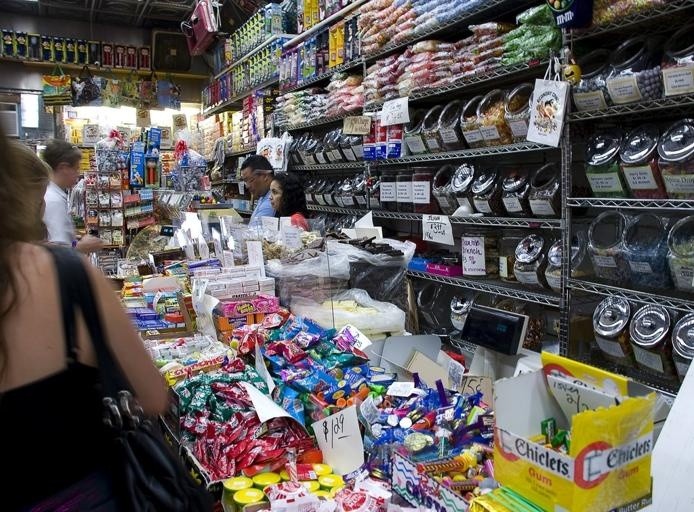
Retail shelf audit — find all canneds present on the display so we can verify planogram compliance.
[223,462,345,512]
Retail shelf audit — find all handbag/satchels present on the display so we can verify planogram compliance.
[99,388,205,512]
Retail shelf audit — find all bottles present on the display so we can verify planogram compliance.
[404,25,693,378]
[286,125,363,237]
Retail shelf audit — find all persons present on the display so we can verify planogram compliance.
[0,137,175,512]
[268,173,308,234]
[240,155,276,230]
[40,138,105,257]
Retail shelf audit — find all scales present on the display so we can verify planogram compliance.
[192,203,245,223]
